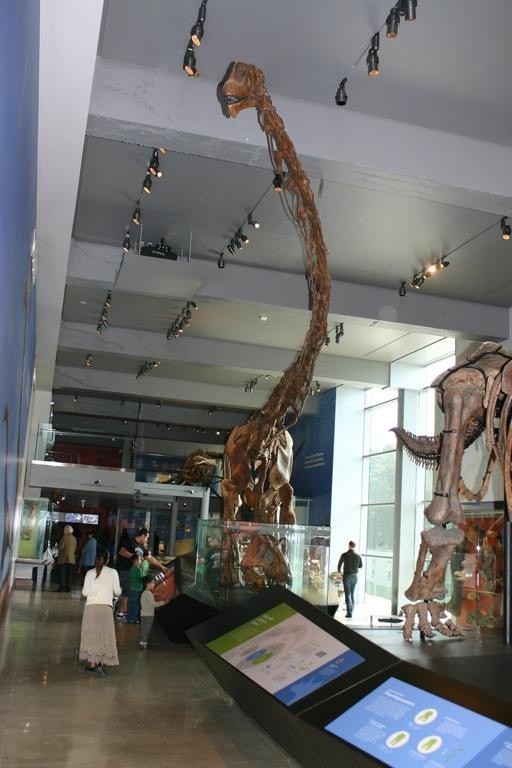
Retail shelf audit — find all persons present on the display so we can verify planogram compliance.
[51,519,170,677]
[337,540,364,618]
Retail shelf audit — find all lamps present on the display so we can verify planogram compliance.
[398,216,511,298]
[207,409,215,416]
[120,148,163,253]
[72,394,78,402]
[164,424,173,431]
[136,359,166,383]
[86,356,93,370]
[119,400,126,406]
[123,418,129,425]
[244,375,269,395]
[164,302,201,345]
[324,322,346,347]
[334,0,417,106]
[195,427,208,435]
[153,401,164,410]
[93,295,114,338]
[309,384,323,397]
[182,1,219,78]
[215,429,233,436]
[217,171,288,271]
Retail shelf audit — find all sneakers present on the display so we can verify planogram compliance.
[85,667,95,672]
[98,667,106,675]
